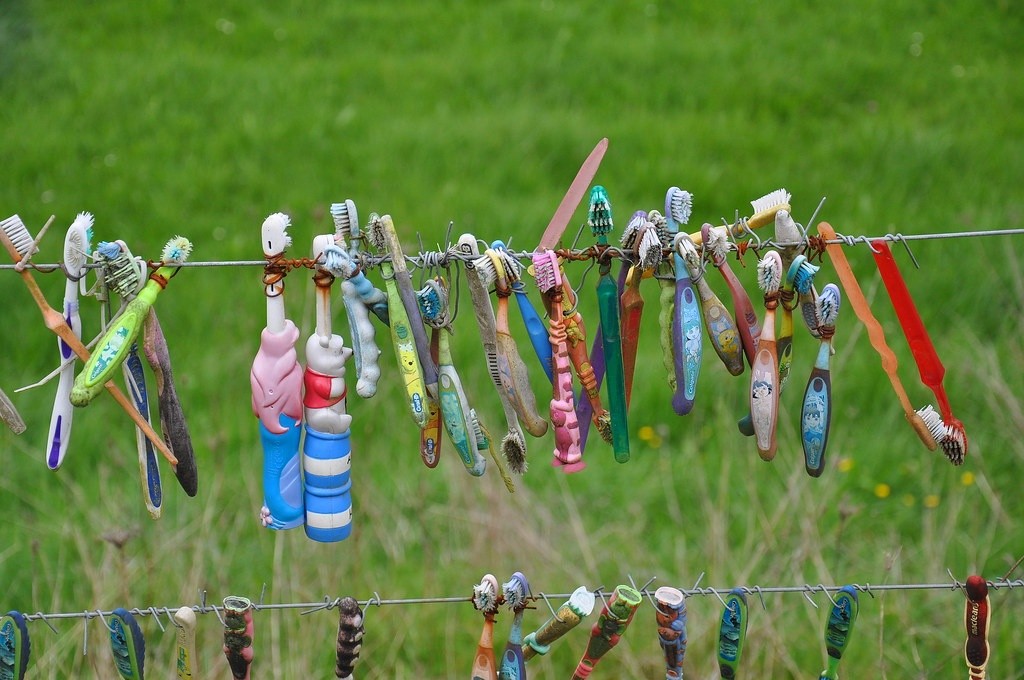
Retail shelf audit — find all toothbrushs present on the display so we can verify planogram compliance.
[1,572,992,680]
[0,212,198,521]
[246,142,970,544]
